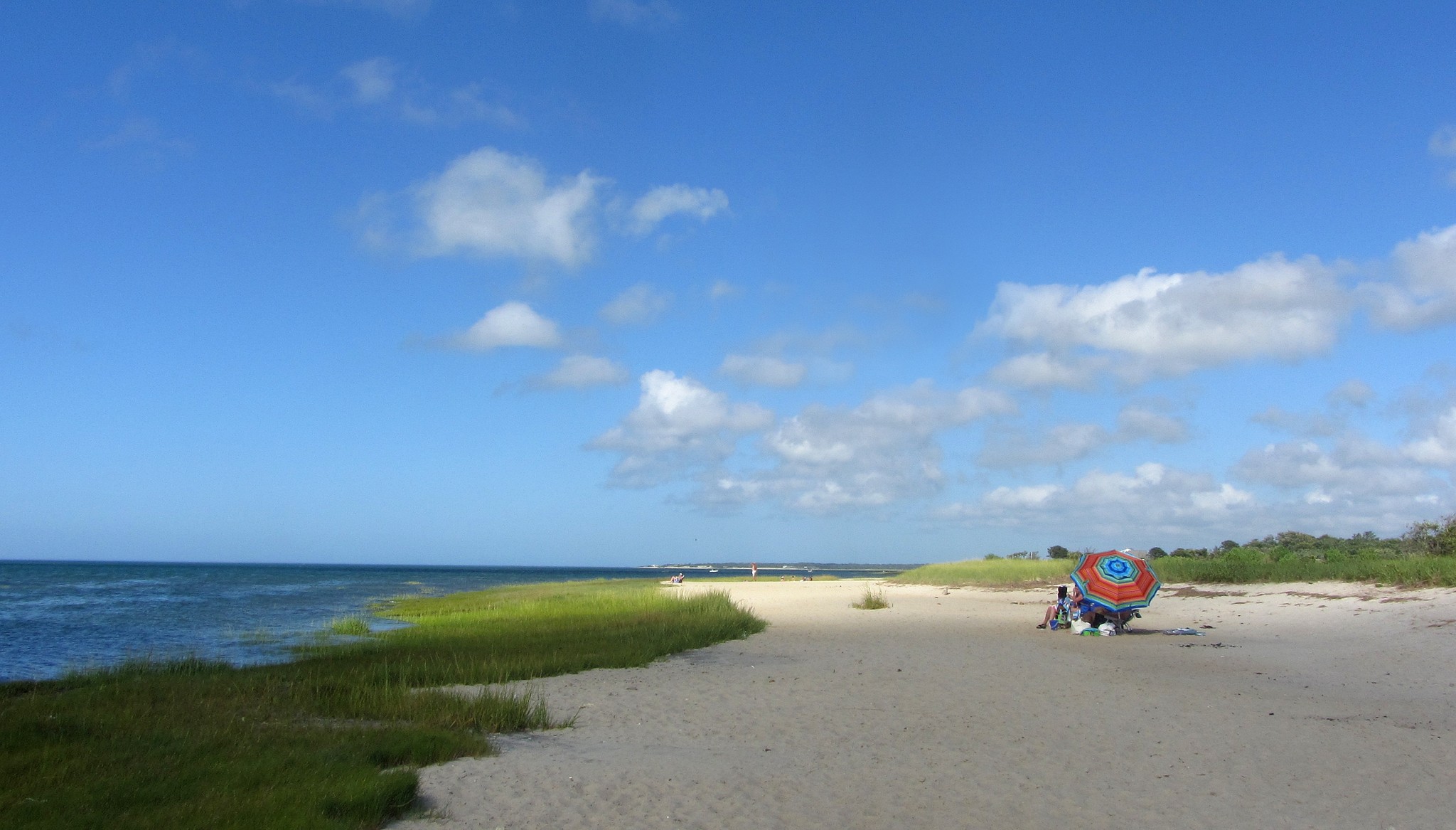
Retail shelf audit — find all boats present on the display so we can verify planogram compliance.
[709,569,719,573]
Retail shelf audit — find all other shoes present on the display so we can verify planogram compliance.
[1037,624,1046,629]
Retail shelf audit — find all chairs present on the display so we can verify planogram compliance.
[1064,594,1140,633]
[674,575,685,583]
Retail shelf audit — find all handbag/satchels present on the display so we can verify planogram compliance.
[1070,619,1091,635]
[1097,623,1117,636]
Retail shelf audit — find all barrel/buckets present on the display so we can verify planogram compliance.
[1050,620,1059,631]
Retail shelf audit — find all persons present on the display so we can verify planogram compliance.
[668,573,685,583]
[1037,584,1131,628]
[750,562,758,582]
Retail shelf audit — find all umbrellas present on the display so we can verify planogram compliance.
[1070,549,1162,632]
